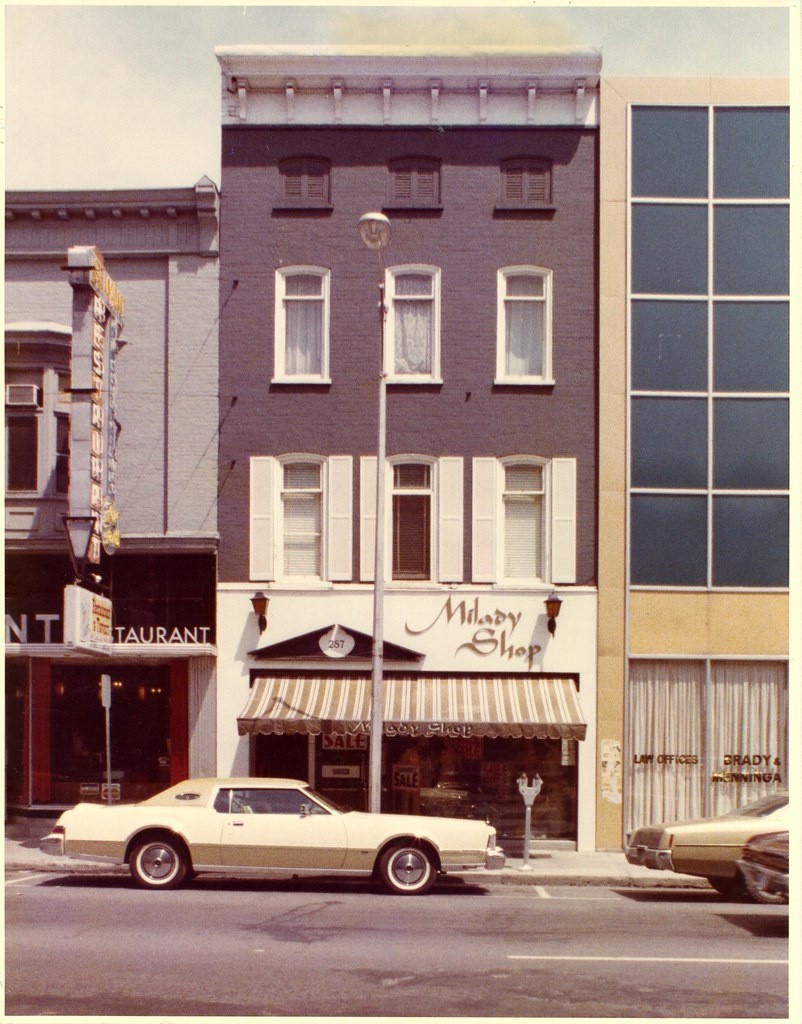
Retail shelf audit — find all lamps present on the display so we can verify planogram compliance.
[250,590,270,633]
[544,586,563,635]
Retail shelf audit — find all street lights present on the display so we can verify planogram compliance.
[355,211,398,812]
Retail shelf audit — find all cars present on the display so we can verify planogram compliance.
[52,777,507,892]
[625,789,787,901]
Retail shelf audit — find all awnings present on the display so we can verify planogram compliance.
[236,672,588,743]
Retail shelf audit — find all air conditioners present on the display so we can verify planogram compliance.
[5,383,44,407]
